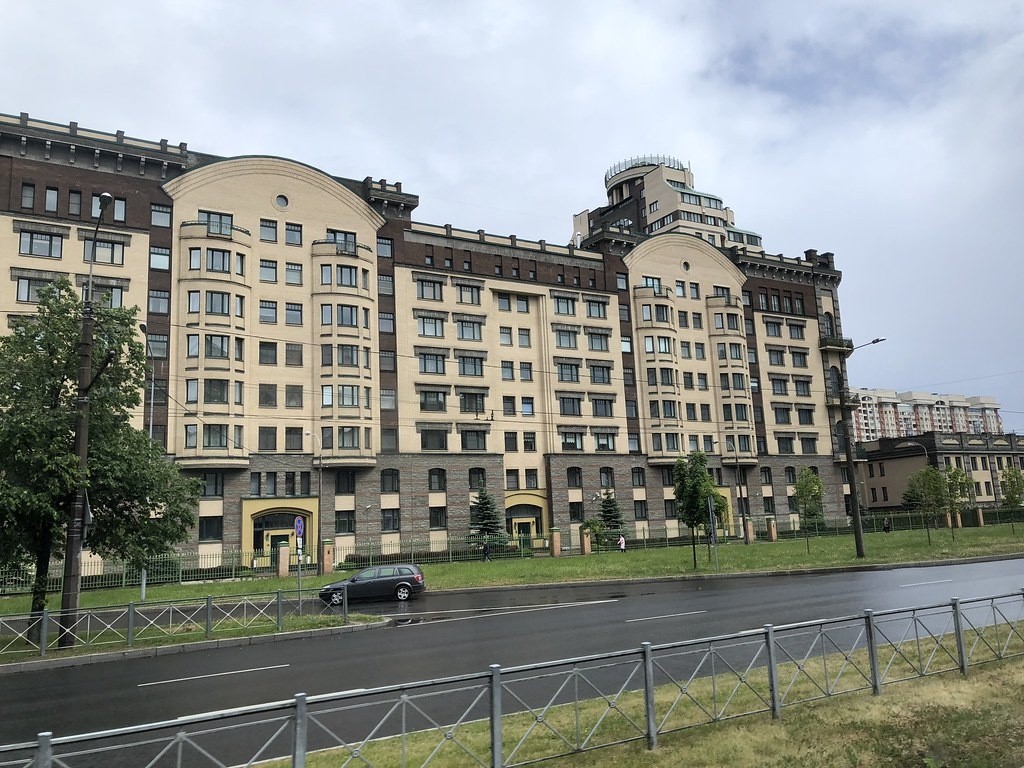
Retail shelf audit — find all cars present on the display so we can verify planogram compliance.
[319,564,425,605]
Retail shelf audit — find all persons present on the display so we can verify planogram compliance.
[883,517,890,533]
[617,534,626,553]
[483,541,492,562]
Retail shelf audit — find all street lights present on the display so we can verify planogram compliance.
[138,324,155,602]
[305,431,323,572]
[908,443,928,467]
[58,192,113,644]
[839,338,887,558]
[713,440,748,544]
